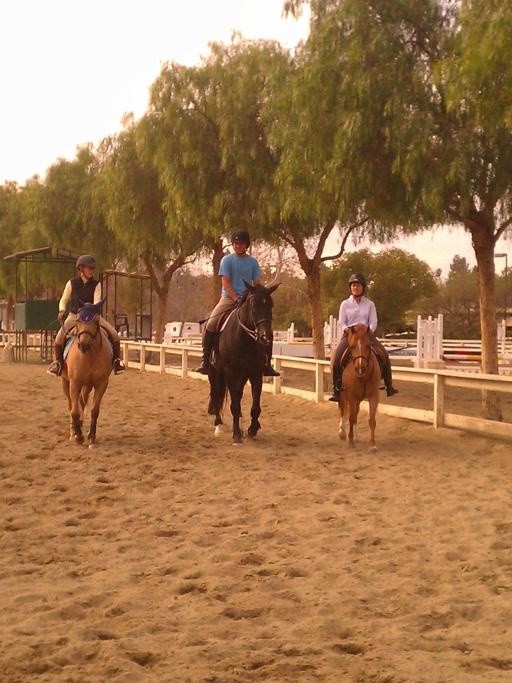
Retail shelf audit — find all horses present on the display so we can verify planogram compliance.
[206,279,283,448]
[329,322,382,453]
[52,292,114,449]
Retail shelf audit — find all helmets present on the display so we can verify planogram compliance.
[348,273,365,284]
[76,255,95,270]
[231,230,249,243]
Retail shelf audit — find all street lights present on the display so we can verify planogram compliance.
[494,253,509,354]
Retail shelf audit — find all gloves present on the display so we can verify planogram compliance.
[57,310,67,325]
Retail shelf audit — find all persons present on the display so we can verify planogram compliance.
[49,255,126,376]
[328,272,400,402]
[196,228,281,377]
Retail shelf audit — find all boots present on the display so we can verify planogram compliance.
[112,341,125,375]
[329,363,342,401]
[381,360,399,396]
[49,345,63,376]
[264,344,280,376]
[196,329,215,373]
